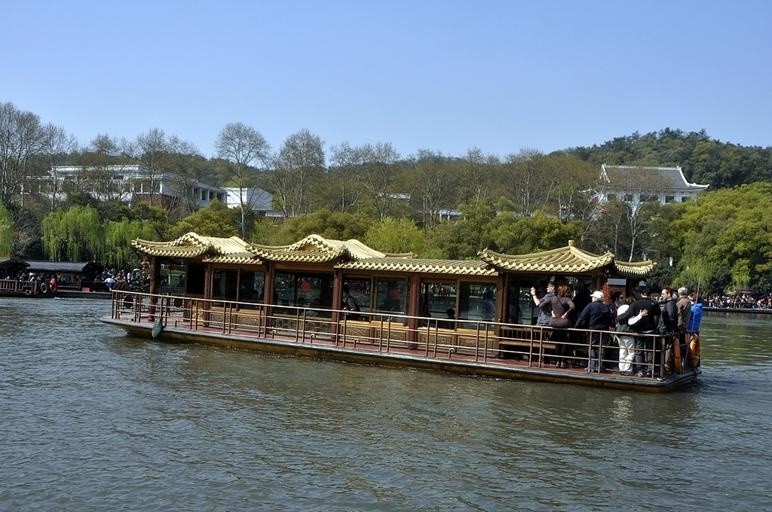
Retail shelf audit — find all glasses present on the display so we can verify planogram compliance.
[620,297,624,299]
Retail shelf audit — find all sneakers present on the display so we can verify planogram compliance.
[585,367,595,373]
[561,362,569,368]
[555,361,561,368]
[595,367,605,372]
[634,368,672,377]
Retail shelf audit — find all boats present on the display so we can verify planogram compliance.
[97,231,701,397]
[20,259,122,300]
[0,255,59,298]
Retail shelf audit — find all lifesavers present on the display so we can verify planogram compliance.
[675,339,685,373]
[40,283,48,293]
[49,280,57,293]
[689,335,700,368]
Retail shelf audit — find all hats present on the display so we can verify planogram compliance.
[678,287,689,295]
[590,290,604,299]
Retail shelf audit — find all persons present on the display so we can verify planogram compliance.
[479,286,531,332]
[343,278,369,321]
[530,281,702,376]
[274,276,333,318]
[261,280,264,299]
[701,293,771,309]
[379,281,401,322]
[420,284,455,330]
[1,260,186,294]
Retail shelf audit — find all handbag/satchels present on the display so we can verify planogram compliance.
[549,317,570,329]
